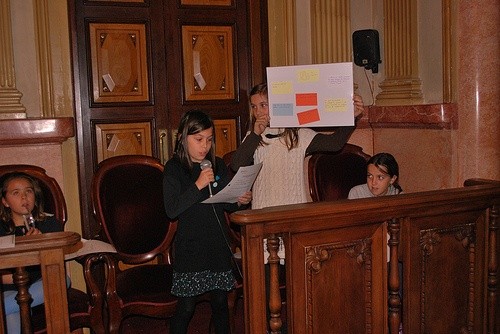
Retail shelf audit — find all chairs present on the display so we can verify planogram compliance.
[308,142,402,205]
[0,163,106,334]
[91,153,237,334]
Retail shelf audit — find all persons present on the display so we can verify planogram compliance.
[0,172,71,334]
[348,153,403,334]
[229,81,364,334]
[163,111,253,334]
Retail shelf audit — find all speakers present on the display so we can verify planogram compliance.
[352,29,380,70]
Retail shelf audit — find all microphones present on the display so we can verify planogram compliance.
[200,160,214,198]
